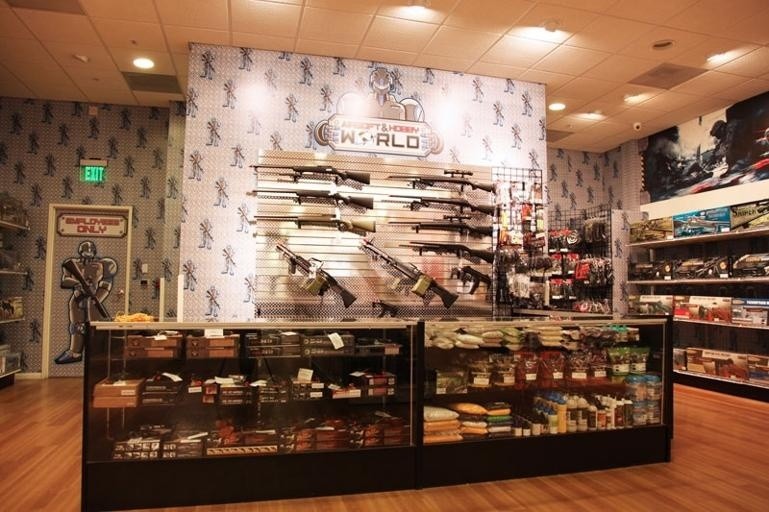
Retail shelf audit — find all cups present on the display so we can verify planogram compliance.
[532,422,544,436]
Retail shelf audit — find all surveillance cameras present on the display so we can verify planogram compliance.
[634,122,642,131]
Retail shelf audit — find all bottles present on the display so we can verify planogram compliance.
[533,392,635,435]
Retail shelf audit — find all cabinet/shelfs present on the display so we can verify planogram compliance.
[0,220,30,391]
[623,179,769,399]
[81,311,673,512]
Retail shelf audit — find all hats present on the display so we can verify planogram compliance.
[709,120,724,137]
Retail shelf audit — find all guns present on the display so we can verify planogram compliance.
[389,193,500,219]
[250,164,370,186]
[371,299,399,316]
[254,213,376,234]
[461,266,490,294]
[253,188,373,211]
[400,239,493,268]
[363,240,458,309]
[64,259,113,321]
[388,168,497,198]
[277,244,356,308]
[388,212,492,239]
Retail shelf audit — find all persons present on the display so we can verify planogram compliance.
[55,241,114,364]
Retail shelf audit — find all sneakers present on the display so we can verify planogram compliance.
[55,349,82,364]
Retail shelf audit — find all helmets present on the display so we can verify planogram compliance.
[368,67,393,87]
[78,241,96,254]
